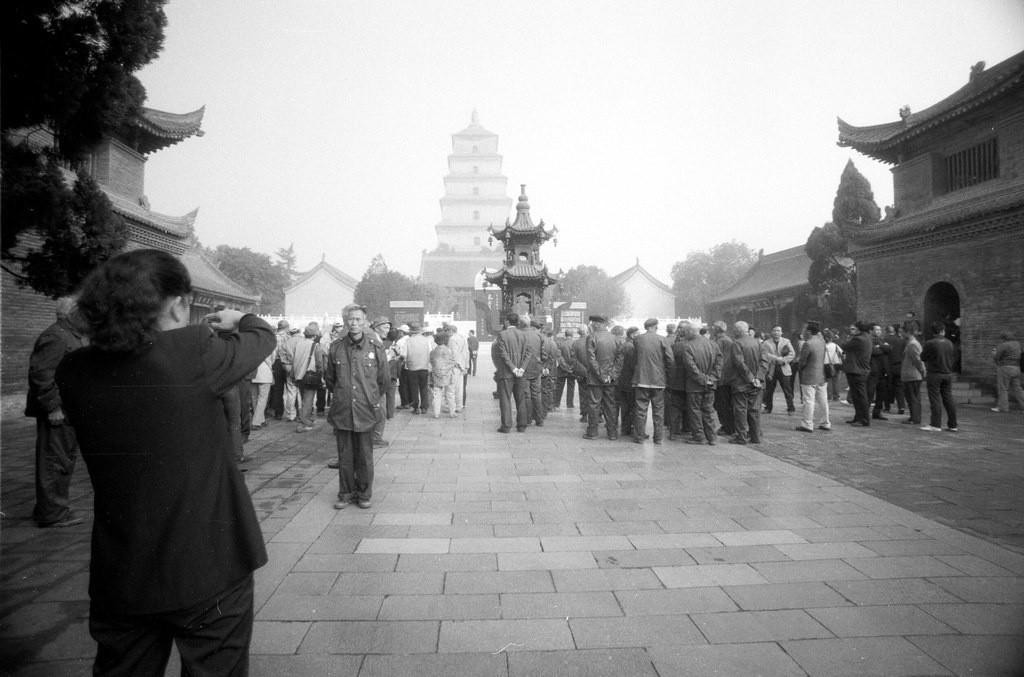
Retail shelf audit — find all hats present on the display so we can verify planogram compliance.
[644,320,657,328]
[444,325,456,332]
[372,317,391,329]
[589,314,607,322]
[397,324,410,333]
[303,326,318,337]
[408,323,422,333]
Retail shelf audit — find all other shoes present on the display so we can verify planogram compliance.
[992,405,1008,413]
[397,399,956,444]
[297,424,315,433]
[327,459,342,467]
[358,496,371,506]
[49,511,84,527]
[251,421,268,430]
[372,438,387,448]
[334,496,354,509]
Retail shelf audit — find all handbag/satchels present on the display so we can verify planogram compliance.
[823,363,836,379]
[302,371,321,389]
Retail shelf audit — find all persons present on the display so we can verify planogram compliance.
[759,324,797,416]
[52,248,275,677]
[323,303,391,510]
[368,314,478,449]
[919,321,959,432]
[790,318,862,433]
[488,313,765,445]
[989,329,1024,414]
[840,321,871,426]
[23,294,90,528]
[867,312,926,424]
[212,303,343,429]
